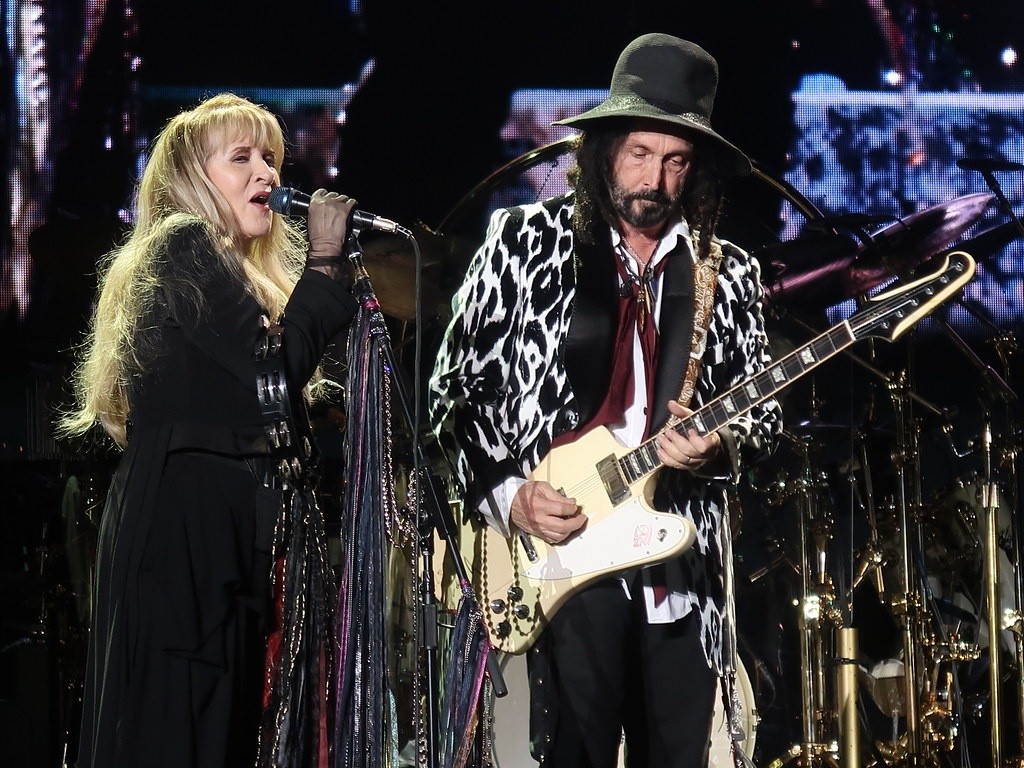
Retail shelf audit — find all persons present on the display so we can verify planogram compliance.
[425,28,785,767]
[70,86,359,767]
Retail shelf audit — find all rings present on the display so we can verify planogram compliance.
[685,457,690,464]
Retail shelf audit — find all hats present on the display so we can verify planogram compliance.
[550,32,754,180]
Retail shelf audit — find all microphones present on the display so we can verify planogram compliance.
[269,186,413,239]
[956,158,1024,171]
[805,213,895,231]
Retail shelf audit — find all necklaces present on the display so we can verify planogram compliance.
[617,234,652,274]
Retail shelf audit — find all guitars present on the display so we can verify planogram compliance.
[412,249,979,657]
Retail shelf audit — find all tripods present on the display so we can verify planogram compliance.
[764,428,842,768]
